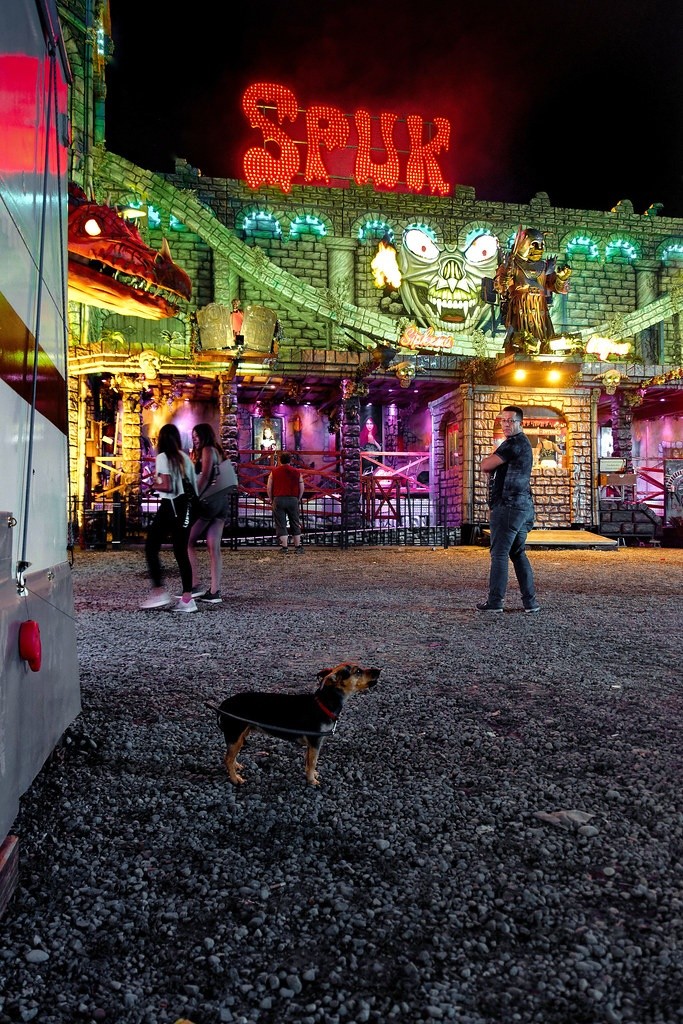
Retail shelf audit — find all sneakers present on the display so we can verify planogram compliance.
[169,598,198,612]
[140,590,172,608]
[174,582,206,598]
[200,587,223,603]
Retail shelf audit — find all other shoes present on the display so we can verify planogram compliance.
[282,546,289,553]
[294,547,304,554]
[475,602,502,613]
[523,606,541,613]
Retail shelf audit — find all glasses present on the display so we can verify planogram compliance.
[499,419,520,424]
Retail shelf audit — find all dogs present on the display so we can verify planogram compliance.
[216,662,381,788]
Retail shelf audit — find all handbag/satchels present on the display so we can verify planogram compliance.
[152,473,173,493]
[193,447,238,505]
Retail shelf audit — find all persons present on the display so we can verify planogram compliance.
[536,435,563,469]
[174,423,230,603]
[229,299,243,335]
[260,428,276,457]
[494,228,571,355]
[267,452,305,554]
[139,424,198,613]
[475,406,540,613]
